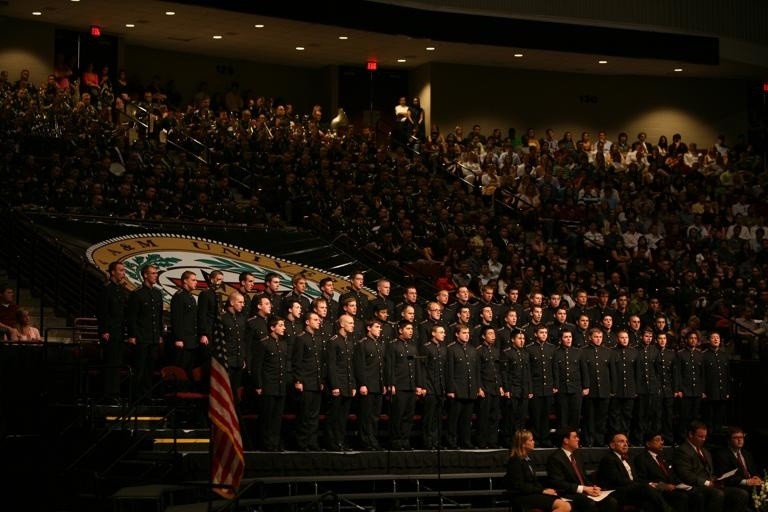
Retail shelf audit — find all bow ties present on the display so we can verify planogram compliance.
[620,454,628,461]
[528,460,535,468]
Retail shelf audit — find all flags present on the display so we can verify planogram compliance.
[205,295,247,500]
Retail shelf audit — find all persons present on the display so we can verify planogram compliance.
[597,433,674,512]
[545,427,619,511]
[674,419,748,512]
[1,69,767,450]
[504,426,574,511]
[716,424,768,512]
[634,431,704,512]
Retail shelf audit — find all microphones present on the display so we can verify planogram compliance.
[408,355,432,360]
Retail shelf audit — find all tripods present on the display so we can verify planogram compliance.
[420,360,463,512]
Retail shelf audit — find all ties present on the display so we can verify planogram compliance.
[656,447,750,479]
[569,454,584,485]
